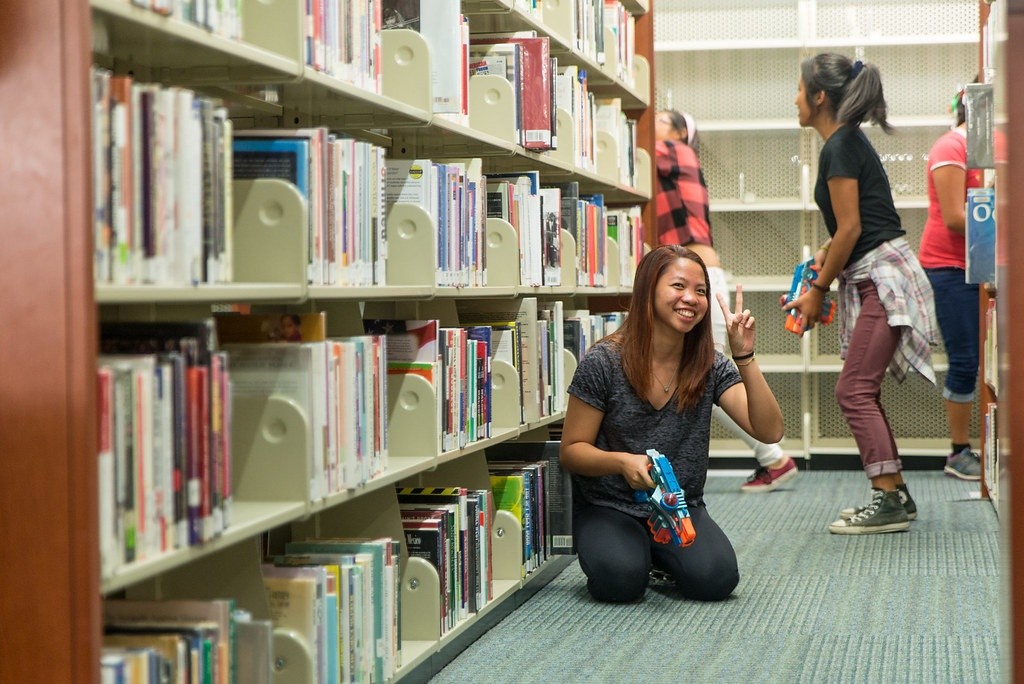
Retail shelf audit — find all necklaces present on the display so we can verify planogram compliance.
[655,363,678,391]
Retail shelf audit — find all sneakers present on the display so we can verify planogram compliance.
[828,484,917,535]
[943,448,981,480]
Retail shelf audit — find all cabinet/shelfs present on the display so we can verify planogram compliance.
[0,0,655,684]
[653,0,979,471]
[980,1,999,518]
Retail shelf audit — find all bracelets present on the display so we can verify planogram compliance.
[820,246,828,251]
[732,351,754,360]
[737,358,755,366]
[813,282,831,293]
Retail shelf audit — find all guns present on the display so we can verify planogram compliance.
[631,448,697,547]
[779,256,836,335]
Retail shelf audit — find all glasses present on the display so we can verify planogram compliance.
[655,116,675,127]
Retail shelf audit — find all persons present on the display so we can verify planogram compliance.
[653,108,798,493]
[778,53,939,534]
[919,78,985,481]
[560,246,785,603]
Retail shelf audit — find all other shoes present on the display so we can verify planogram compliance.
[741,459,796,493]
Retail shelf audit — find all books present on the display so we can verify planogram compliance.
[88,0,652,684]
[961,4,1000,512]
